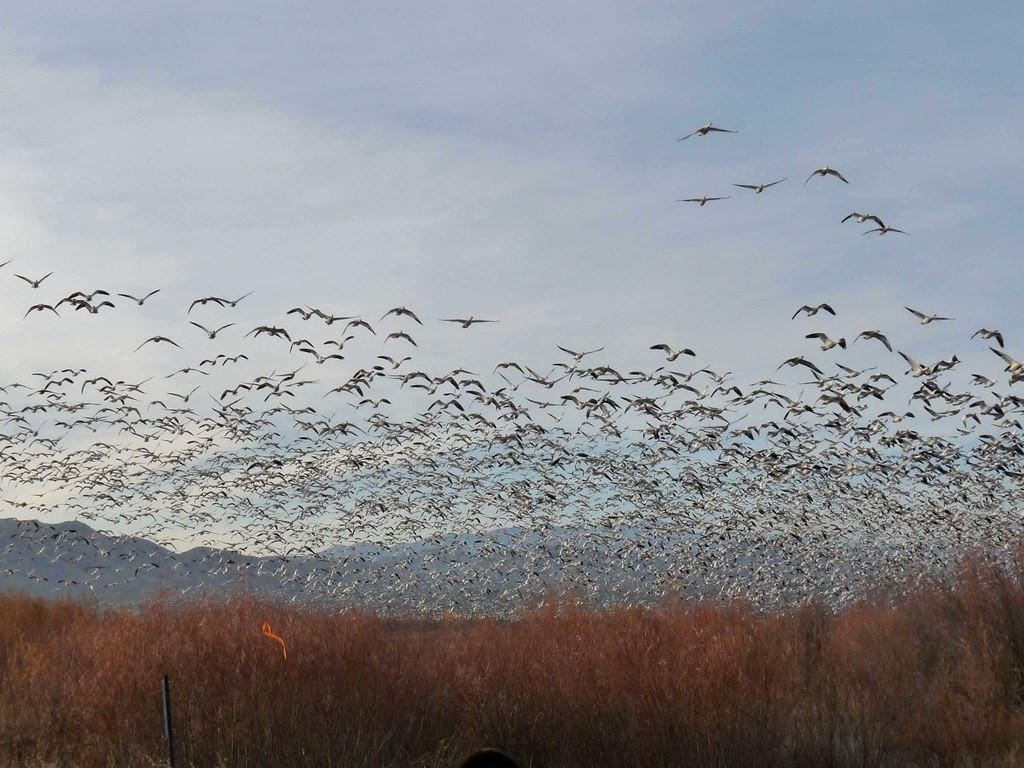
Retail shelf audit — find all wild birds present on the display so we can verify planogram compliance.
[0,258,1024,620]
[733,176,791,194]
[677,122,739,141]
[677,194,731,206]
[804,166,850,185]
[841,212,885,228]
[864,226,910,237]
